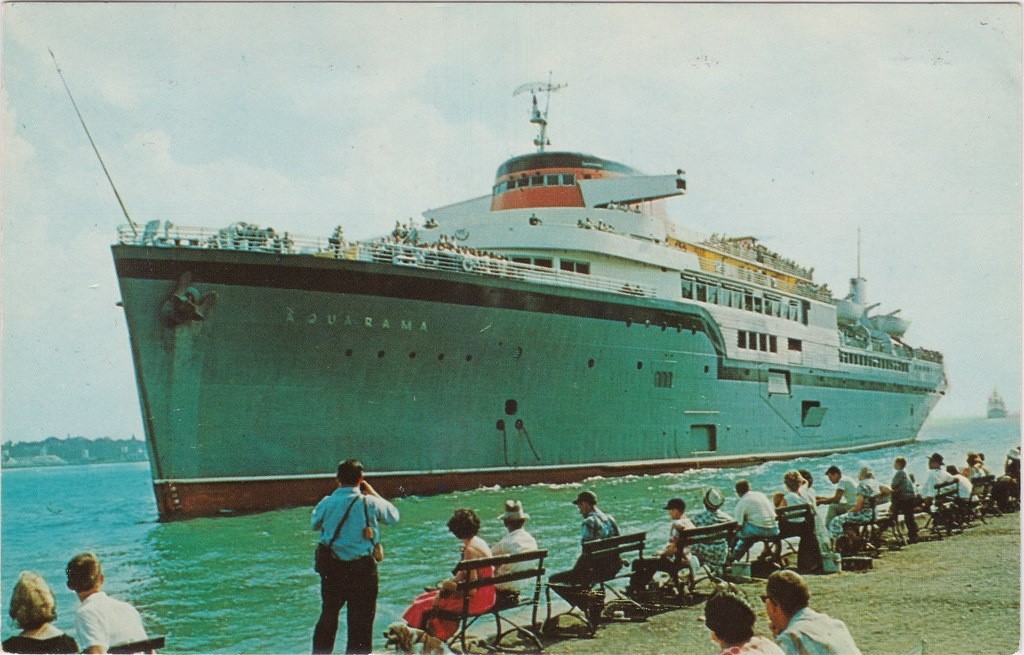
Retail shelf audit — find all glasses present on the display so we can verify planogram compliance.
[761,592,773,603]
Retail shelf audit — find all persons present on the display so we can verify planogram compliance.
[727,469,817,562]
[664,238,687,252]
[548,492,620,619]
[2,571,78,654]
[608,200,642,213]
[578,218,615,233]
[815,446,1021,551]
[209,221,294,251]
[529,213,538,225]
[627,490,733,591]
[706,570,861,655]
[402,501,539,640]
[66,553,155,654]
[619,282,644,296]
[331,218,518,280]
[709,231,833,303]
[843,328,945,363]
[311,460,399,655]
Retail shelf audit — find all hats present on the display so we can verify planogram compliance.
[571,491,597,505]
[496,501,531,520]
[927,452,944,465]
[662,498,686,511]
[703,487,723,508]
[1005,447,1020,460]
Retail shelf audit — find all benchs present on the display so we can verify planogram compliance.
[854,491,904,548]
[963,474,1005,523]
[545,531,648,632]
[669,520,740,588]
[418,548,550,655]
[926,477,974,531]
[758,506,814,569]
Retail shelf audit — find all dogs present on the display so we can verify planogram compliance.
[383,623,464,655]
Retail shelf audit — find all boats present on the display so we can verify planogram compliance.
[986,389,1008,419]
[46,46,948,521]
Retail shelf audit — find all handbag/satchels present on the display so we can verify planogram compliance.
[313,543,336,574]
[822,551,842,573]
